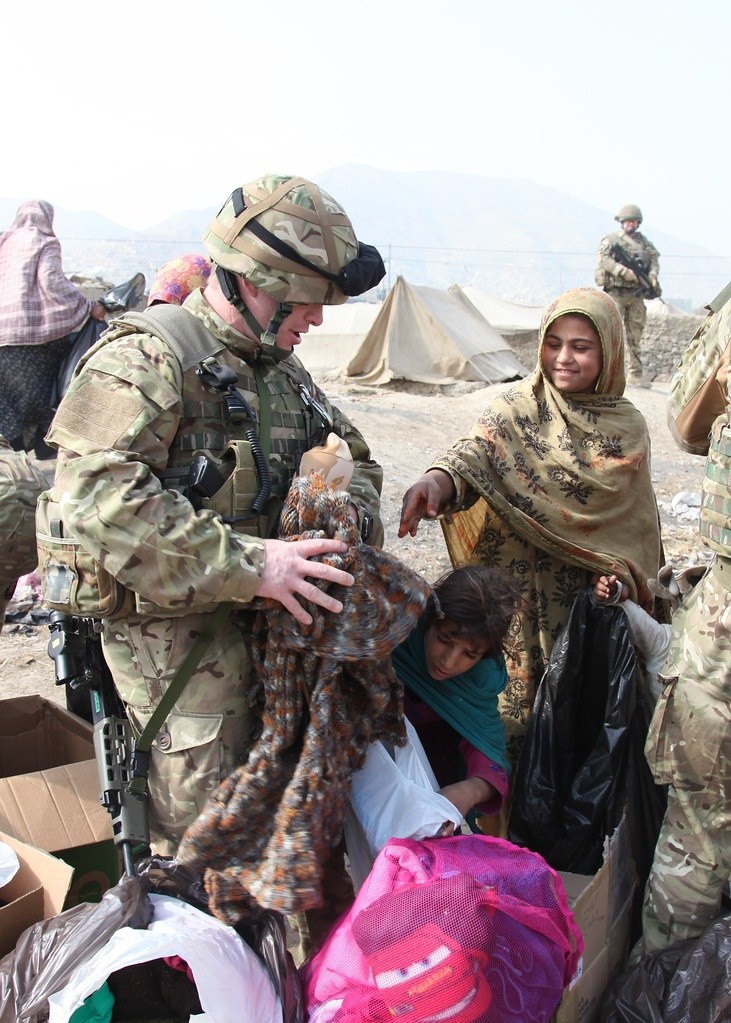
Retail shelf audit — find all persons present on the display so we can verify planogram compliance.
[394,290,663,841]
[42,173,384,855]
[0,198,730,1023]
[596,202,660,377]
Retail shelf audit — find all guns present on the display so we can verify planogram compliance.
[49,608,151,892]
[188,456,227,497]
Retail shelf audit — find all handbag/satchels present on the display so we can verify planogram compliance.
[345,713,465,864]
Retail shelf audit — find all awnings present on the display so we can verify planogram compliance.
[315,275,531,383]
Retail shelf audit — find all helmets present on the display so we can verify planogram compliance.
[202,175,357,305]
[618,204,642,224]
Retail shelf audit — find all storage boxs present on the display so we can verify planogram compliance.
[0,825,76,958]
[0,693,119,903]
[549,805,633,1023]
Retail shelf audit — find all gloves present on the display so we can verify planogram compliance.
[651,279,661,297]
[622,267,638,283]
